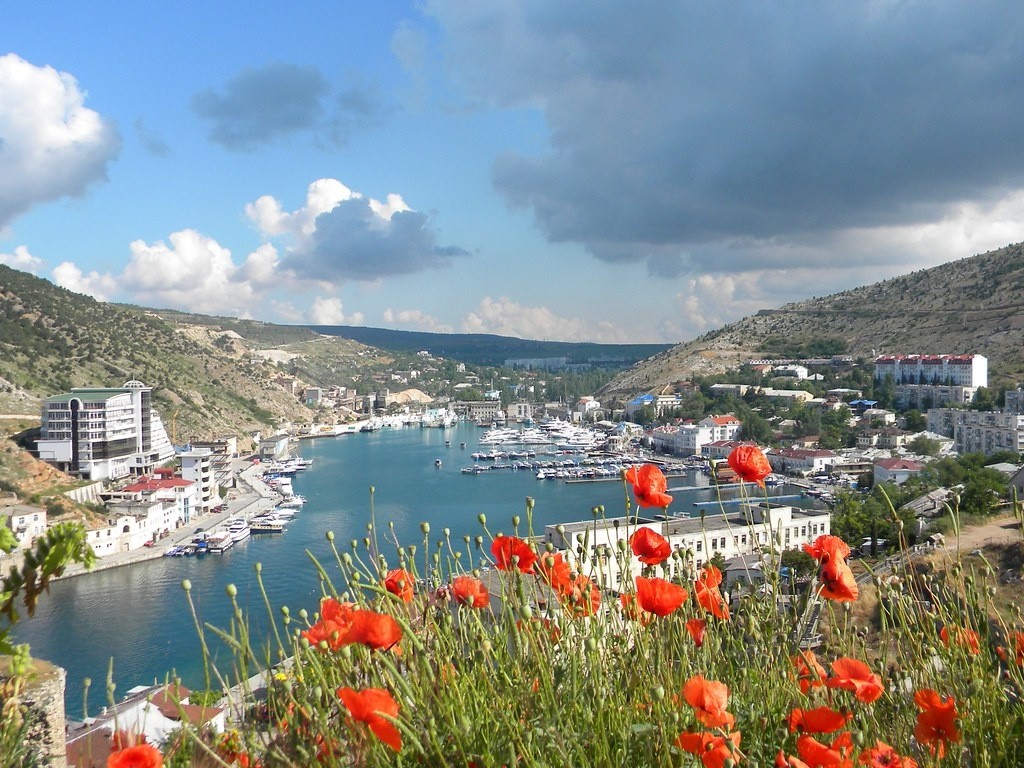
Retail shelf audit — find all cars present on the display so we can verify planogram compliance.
[144,540,155,546]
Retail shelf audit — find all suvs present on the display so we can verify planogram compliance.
[219,504,229,510]
[214,506,222,513]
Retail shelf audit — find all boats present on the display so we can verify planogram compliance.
[471,449,536,460]
[461,441,466,448]
[435,459,441,466]
[478,415,607,455]
[459,457,688,480]
[445,440,450,445]
[688,465,840,505]
[163,454,313,556]
